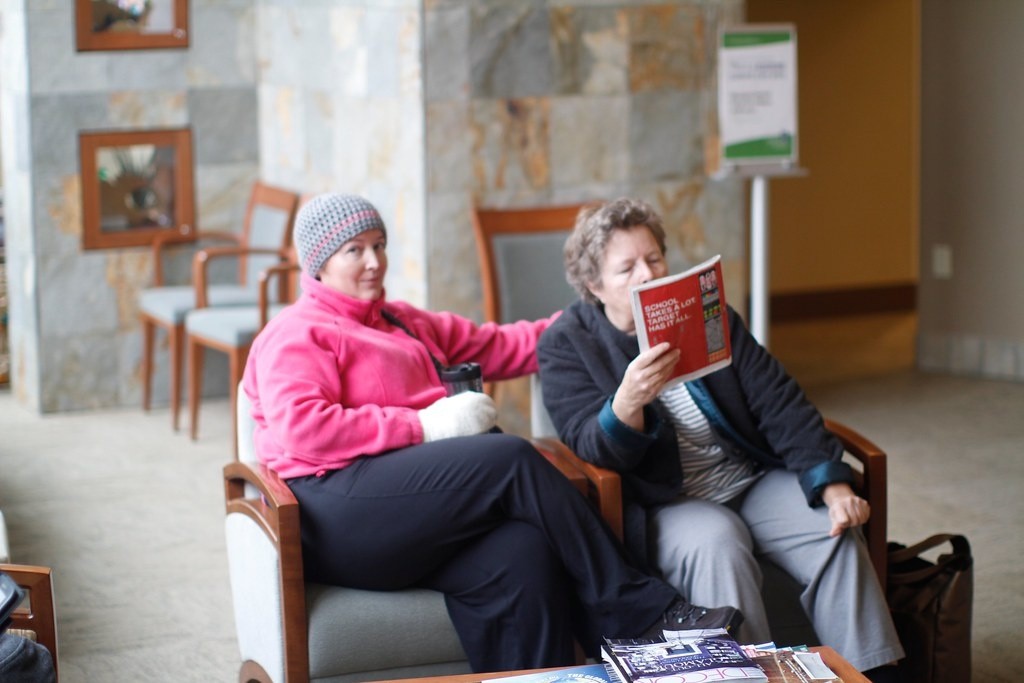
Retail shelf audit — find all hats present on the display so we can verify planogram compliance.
[293,193,386,280]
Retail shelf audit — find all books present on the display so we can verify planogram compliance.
[629,254,732,399]
[600,633,768,682]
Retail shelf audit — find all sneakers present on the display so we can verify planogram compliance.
[636,594,744,639]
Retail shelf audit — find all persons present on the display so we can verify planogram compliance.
[534,196,912,682]
[236,192,745,681]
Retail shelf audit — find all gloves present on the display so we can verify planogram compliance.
[417,391,497,443]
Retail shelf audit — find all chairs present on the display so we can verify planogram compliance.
[137,182,300,431]
[184,196,316,458]
[529,368,888,648]
[471,200,580,400]
[222,378,593,683]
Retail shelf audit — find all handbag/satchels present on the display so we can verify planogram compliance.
[881,534,974,683]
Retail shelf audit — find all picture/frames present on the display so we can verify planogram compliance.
[80,128,197,249]
[75,0,189,52]
